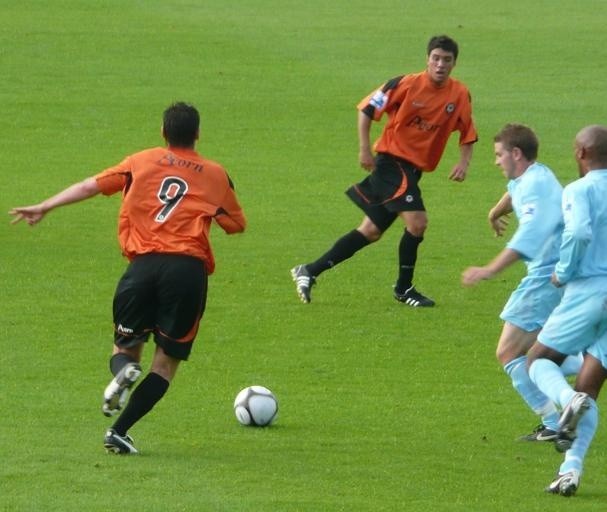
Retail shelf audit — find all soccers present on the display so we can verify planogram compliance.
[234,384,278,428]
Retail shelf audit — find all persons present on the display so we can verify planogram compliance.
[459,122,584,445]
[6,98,247,456]
[289,34,478,309]
[523,125,607,495]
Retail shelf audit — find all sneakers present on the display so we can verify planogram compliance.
[545,471,580,497]
[554,392,591,453]
[290,263,316,304]
[102,362,144,418]
[393,282,435,307]
[104,427,139,456]
[522,427,560,446]
